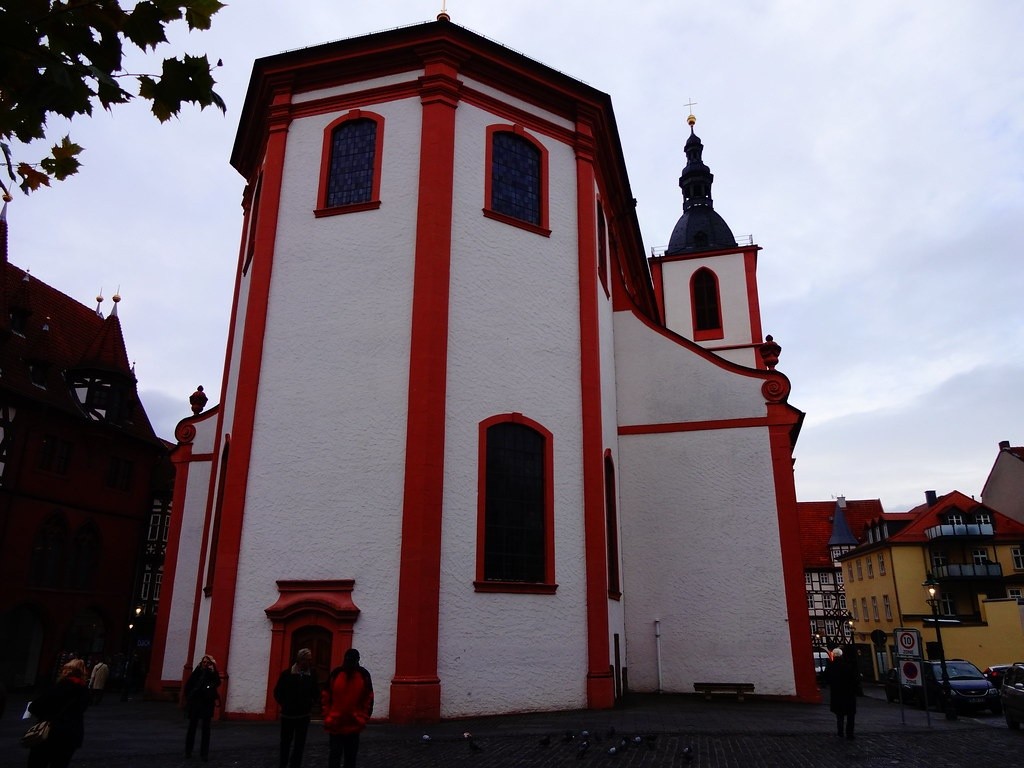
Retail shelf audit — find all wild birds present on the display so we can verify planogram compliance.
[421,730,692,758]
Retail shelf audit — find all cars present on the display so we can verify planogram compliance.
[885,667,923,707]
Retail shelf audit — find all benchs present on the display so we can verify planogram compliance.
[694,682,755,703]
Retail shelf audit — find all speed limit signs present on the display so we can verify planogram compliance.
[897,631,920,657]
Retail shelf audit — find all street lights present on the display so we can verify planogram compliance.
[923,570,957,720]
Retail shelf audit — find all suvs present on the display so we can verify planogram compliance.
[922,659,1002,717]
[983,664,1012,690]
[1001,662,1024,730]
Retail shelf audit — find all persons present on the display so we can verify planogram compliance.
[28,657,88,767]
[180,652,221,766]
[827,645,860,742]
[273,647,323,767]
[320,647,375,767]
[89,656,110,705]
